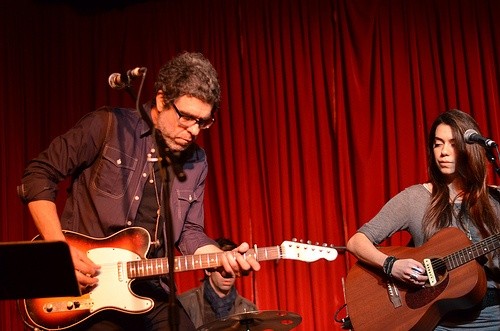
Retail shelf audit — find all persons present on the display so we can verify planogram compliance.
[17,52,260,331]
[176,237,259,331]
[346,110,500,331]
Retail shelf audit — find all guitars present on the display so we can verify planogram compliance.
[17,227,338,331]
[345,226,500,331]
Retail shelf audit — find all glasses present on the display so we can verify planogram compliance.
[165,93,216,130]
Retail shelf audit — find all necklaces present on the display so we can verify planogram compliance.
[447,198,475,241]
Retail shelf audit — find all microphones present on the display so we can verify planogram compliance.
[464,129,498,148]
[108,67,143,90]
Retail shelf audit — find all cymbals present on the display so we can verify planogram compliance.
[196,310,301,331]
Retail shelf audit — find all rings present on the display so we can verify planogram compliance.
[415,267,422,273]
[410,272,418,279]
